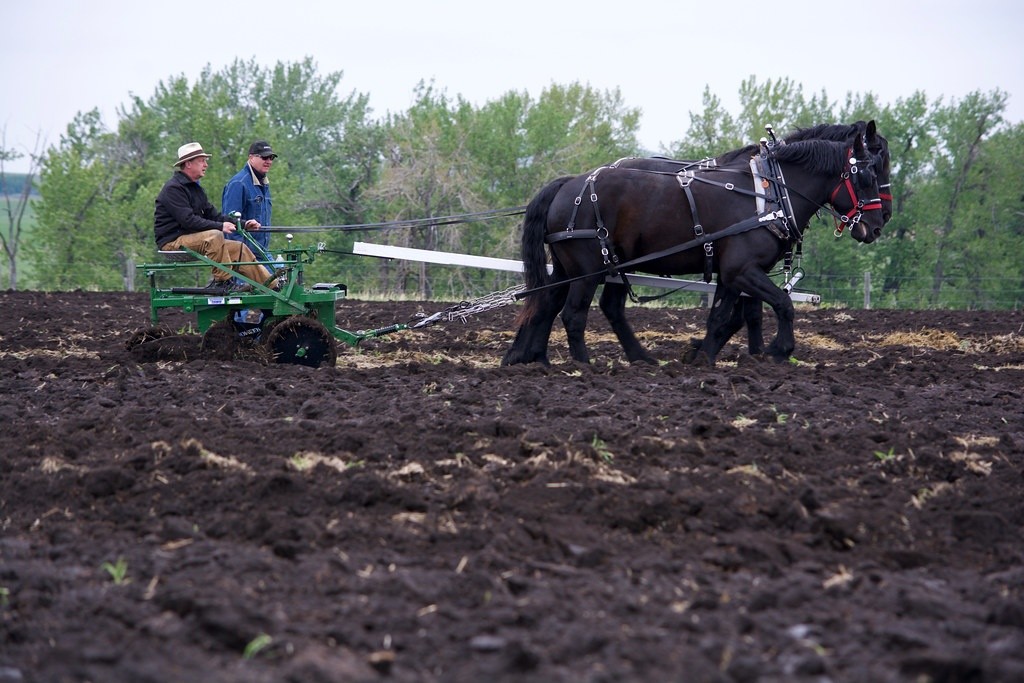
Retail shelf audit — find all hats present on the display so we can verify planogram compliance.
[249,140,278,158]
[173,142,212,167]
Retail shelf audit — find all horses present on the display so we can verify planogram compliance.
[496,120,892,364]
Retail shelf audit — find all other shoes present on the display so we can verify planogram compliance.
[274,277,288,292]
[215,281,250,292]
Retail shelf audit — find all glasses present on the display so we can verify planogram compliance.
[253,154,275,160]
[194,157,209,163]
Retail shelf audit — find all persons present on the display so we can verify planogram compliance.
[153,141,287,293]
[222,141,277,324]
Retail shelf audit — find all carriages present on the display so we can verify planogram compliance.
[131,115,900,373]
